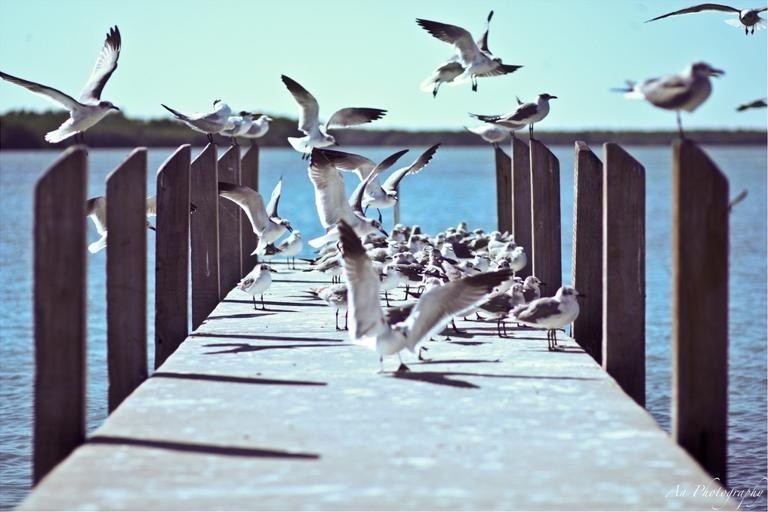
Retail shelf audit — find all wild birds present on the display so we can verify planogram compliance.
[610,58,726,136]
[1,22,127,147]
[215,174,303,311]
[462,91,558,147]
[292,217,581,380]
[161,98,273,148]
[413,8,525,97]
[303,141,442,250]
[279,74,389,161]
[643,1,767,35]
[84,190,198,253]
